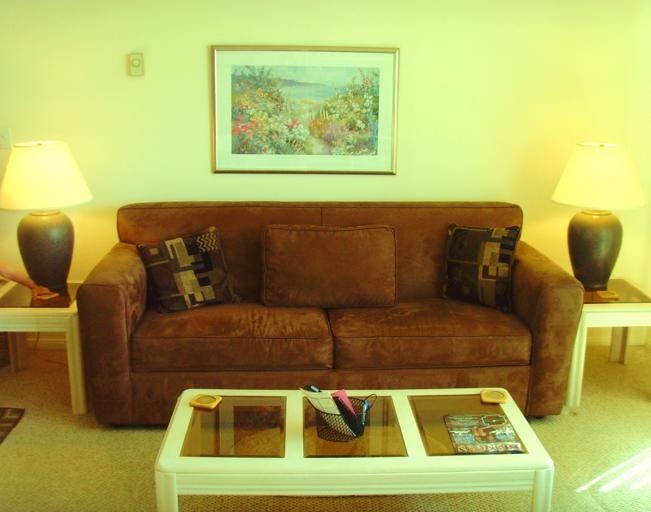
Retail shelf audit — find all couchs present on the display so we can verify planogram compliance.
[76,202,586,427]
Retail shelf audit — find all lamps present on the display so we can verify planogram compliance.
[551,140,644,292]
[0,140,92,289]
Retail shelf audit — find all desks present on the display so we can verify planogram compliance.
[565,279,651,407]
[0,279,87,416]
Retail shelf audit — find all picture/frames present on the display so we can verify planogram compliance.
[212,45,400,175]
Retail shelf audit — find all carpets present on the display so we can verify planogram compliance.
[1,408,24,445]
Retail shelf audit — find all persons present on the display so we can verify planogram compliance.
[0,261,53,301]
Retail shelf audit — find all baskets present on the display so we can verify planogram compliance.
[304,393,378,443]
